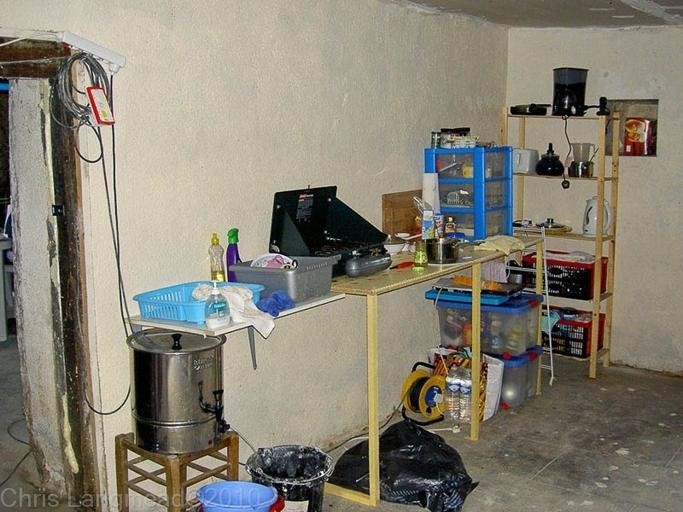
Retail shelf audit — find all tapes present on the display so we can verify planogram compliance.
[412,266,425,272]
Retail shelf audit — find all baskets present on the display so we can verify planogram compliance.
[522,250,608,300]
[540,305,606,358]
[133,280,265,326]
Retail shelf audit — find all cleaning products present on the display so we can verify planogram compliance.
[227,229,239,282]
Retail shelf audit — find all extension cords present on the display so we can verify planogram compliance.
[62,31,125,68]
[87,86,115,126]
[453,274,500,291]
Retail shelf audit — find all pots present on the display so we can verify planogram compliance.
[426,238,461,264]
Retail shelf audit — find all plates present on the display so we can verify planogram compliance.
[251,254,300,270]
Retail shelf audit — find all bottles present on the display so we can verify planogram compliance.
[535,143,565,176]
[414,238,428,269]
[444,364,472,427]
[462,155,474,178]
[430,127,476,150]
[433,216,456,239]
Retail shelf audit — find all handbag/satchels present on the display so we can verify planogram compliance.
[427,348,505,424]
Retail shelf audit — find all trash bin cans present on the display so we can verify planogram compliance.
[245,445,334,512]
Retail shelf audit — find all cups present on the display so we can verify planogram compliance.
[485,158,493,179]
[570,143,595,163]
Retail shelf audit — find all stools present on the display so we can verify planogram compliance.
[116,431,239,512]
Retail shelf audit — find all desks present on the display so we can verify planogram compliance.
[0,239,15,342]
[323,237,543,506]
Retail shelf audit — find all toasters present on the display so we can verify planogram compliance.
[512,148,538,174]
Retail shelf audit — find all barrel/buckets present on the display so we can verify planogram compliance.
[195,482,278,510]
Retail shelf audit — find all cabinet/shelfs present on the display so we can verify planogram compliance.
[425,148,512,243]
[501,106,619,379]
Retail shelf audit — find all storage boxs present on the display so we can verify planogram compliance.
[489,346,542,409]
[436,295,543,356]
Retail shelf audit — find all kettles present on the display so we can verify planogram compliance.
[583,196,612,238]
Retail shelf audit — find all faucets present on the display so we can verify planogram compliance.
[204,390,230,434]
